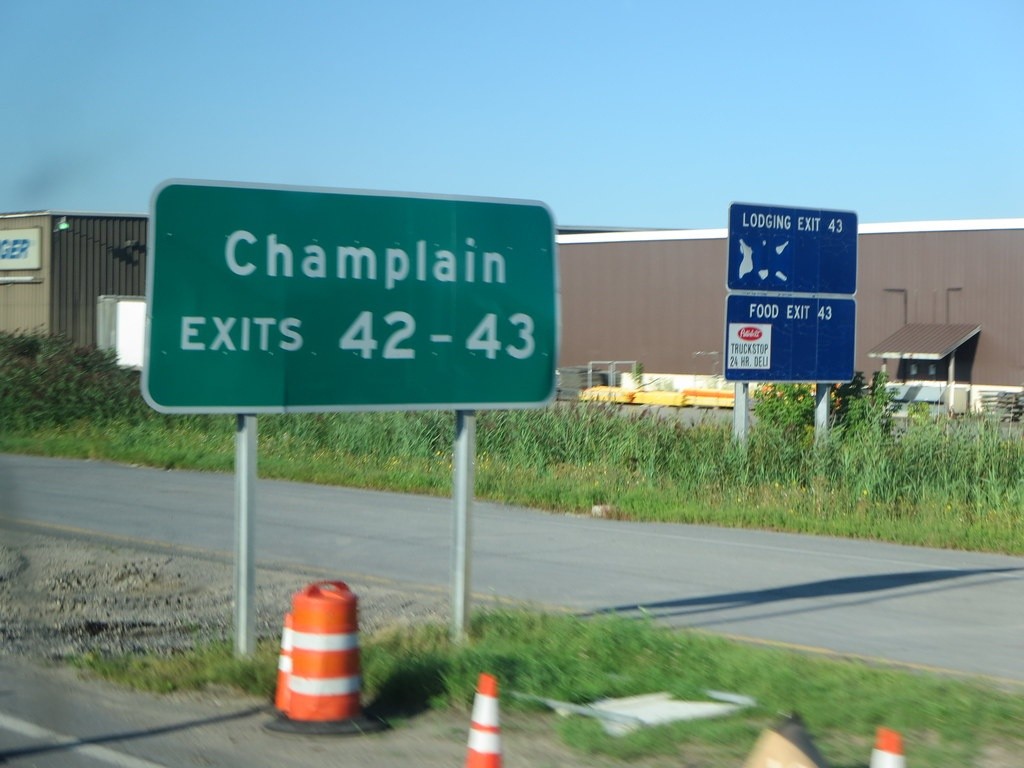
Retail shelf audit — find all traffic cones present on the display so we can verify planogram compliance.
[460,670,502,768]
